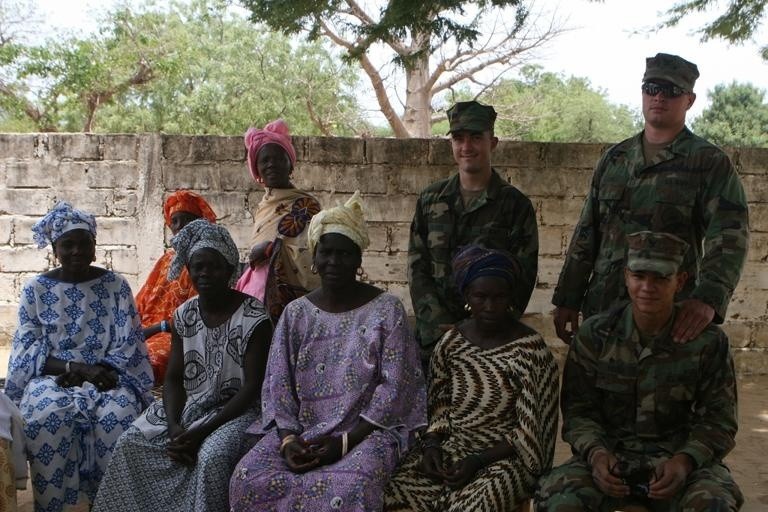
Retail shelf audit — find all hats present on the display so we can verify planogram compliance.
[643,51,698,92]
[441,100,498,134]
[624,227,689,279]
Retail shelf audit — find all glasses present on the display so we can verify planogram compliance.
[639,82,692,98]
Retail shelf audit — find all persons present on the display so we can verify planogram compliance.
[233,119,321,329]
[551,52,749,344]
[383,246,561,512]
[132,192,215,387]
[91,218,277,511]
[229,189,427,511]
[407,101,541,374]
[2,198,154,510]
[532,226,745,512]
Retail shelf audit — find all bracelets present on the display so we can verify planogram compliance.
[160,320,167,332]
[341,432,348,458]
[66,360,73,375]
[264,242,273,257]
[279,434,295,453]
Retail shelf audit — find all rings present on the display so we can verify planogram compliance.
[64,381,70,386]
[99,382,103,386]
